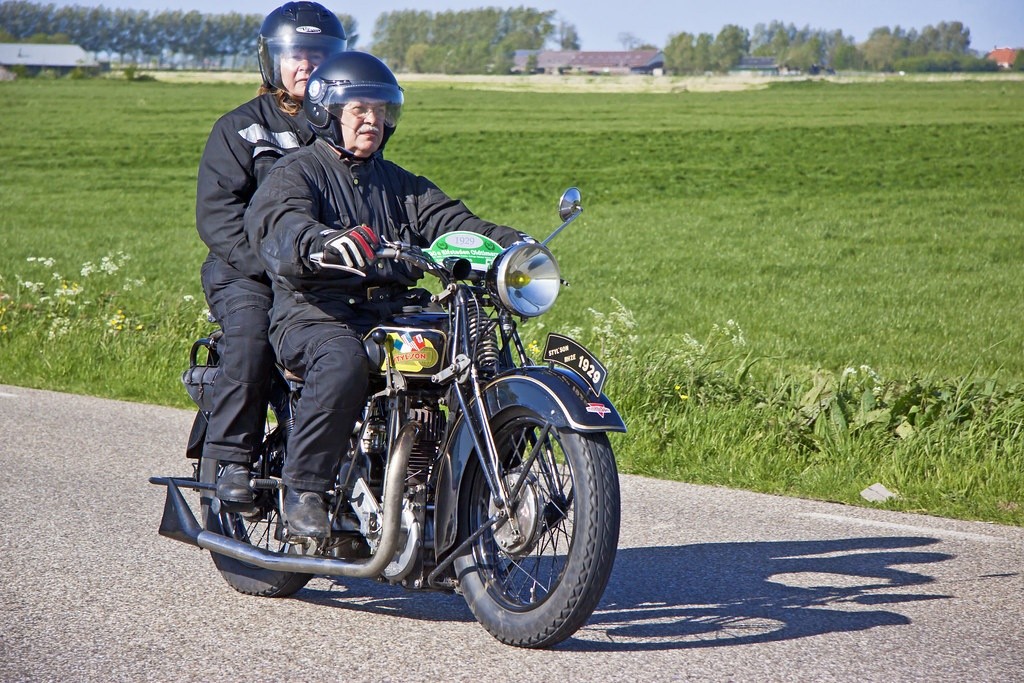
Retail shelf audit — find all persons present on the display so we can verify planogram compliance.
[196,2,348,503]
[244,51,538,541]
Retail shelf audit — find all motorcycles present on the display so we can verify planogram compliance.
[149,186,628,649]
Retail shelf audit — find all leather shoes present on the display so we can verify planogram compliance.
[217,463,251,503]
[282,488,330,538]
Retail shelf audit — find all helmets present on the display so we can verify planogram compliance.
[257,1,347,87]
[302,51,404,128]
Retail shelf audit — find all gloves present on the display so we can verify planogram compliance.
[291,224,381,276]
